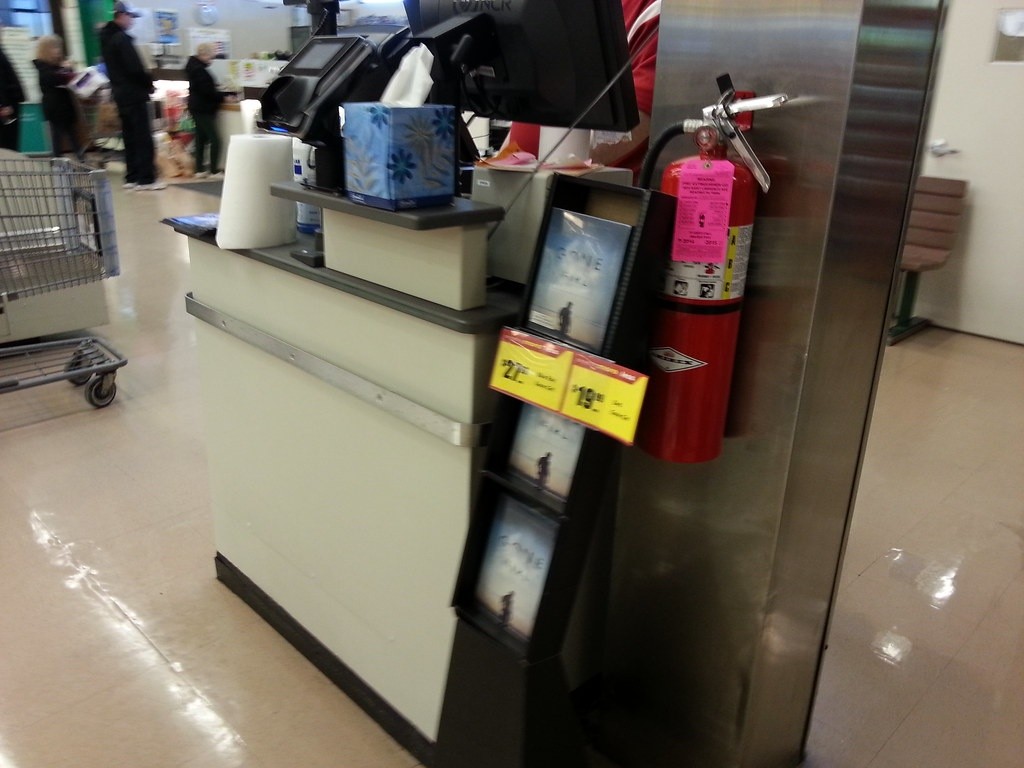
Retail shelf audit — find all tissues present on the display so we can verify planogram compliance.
[339,42,458,211]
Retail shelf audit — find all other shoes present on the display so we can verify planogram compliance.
[135,181,168,191]
[209,171,224,178]
[194,172,208,178]
[122,182,136,189]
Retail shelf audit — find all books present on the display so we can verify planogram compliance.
[519,205,635,361]
[507,398,585,501]
[472,496,559,644]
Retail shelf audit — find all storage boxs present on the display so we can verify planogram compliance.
[54,66,195,178]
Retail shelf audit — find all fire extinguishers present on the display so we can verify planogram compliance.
[639,73,788,463]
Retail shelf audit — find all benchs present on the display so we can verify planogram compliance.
[883,175,973,345]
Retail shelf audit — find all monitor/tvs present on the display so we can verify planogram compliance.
[403,0,641,168]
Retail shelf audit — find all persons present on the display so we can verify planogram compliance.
[101,2,168,191]
[0,48,25,150]
[33,34,89,163]
[185,41,224,176]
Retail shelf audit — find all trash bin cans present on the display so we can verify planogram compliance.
[17,102,47,152]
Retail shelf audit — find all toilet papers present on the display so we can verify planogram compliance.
[537,124,592,167]
[215,132,297,249]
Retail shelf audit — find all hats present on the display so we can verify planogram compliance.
[114,0,143,18]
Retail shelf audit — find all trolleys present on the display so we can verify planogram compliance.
[0,155,130,410]
